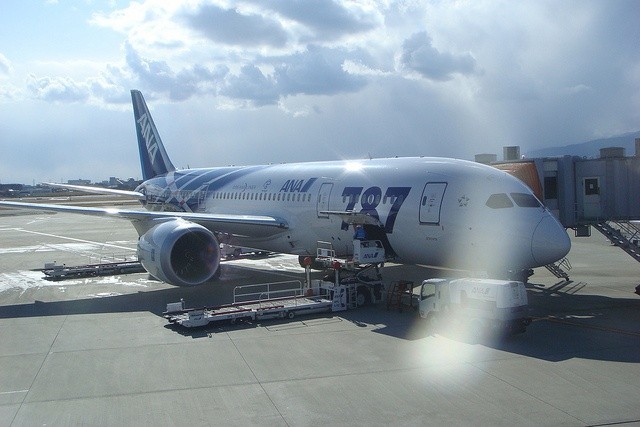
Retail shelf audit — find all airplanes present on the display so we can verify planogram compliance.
[1,89,572,287]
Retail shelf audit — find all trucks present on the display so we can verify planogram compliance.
[418,277,527,335]
[161,240,389,330]
